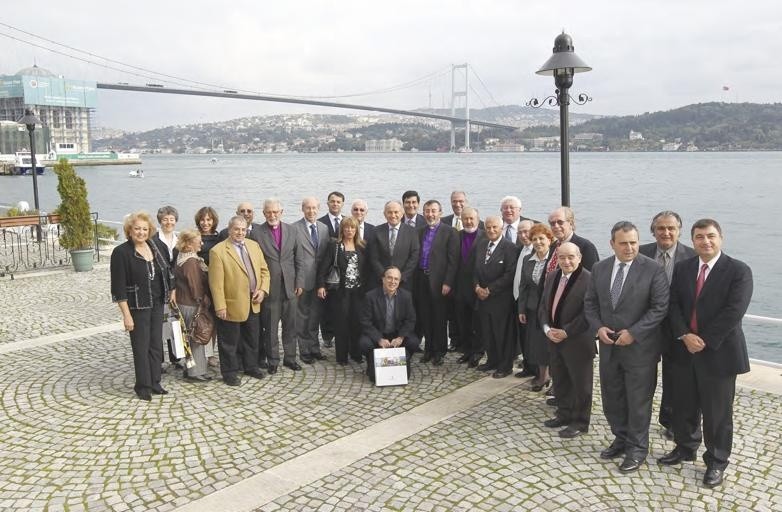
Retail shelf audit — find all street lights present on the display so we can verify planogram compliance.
[15,105,50,245]
[530,28,595,208]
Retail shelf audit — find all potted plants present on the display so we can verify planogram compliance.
[52,157,95,272]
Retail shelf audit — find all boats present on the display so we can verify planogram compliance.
[208,157,218,166]
[127,170,145,178]
[43,142,145,167]
[12,147,46,174]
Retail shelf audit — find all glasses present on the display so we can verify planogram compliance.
[550,220,567,225]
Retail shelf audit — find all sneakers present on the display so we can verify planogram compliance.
[514,372,530,378]
[283,359,302,370]
[457,354,492,370]
[493,368,512,378]
[173,354,218,382]
[222,375,241,386]
[421,350,442,365]
[546,384,557,405]
[300,352,325,364]
[243,361,279,379]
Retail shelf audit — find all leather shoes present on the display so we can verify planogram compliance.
[133,383,167,402]
[703,469,724,486]
[659,448,696,465]
[543,418,567,428]
[560,425,580,439]
[599,447,621,459]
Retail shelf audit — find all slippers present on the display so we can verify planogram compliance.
[620,455,641,472]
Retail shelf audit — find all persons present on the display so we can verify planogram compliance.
[584,220,668,471]
[638,212,696,432]
[658,219,752,487]
[154,189,599,439]
[111,212,177,401]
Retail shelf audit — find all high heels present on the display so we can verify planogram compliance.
[533,379,550,392]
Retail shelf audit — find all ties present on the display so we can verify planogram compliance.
[660,251,668,272]
[388,228,398,257]
[407,218,414,225]
[236,244,256,291]
[689,264,708,331]
[550,277,569,320]
[334,215,339,234]
[454,217,461,232]
[610,262,626,308]
[484,242,496,261]
[309,223,318,249]
[546,243,561,277]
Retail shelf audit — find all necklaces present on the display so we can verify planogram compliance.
[137,245,155,280]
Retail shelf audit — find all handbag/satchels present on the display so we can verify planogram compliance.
[188,300,216,348]
[345,321,372,356]
[372,343,409,387]
[160,302,184,358]
